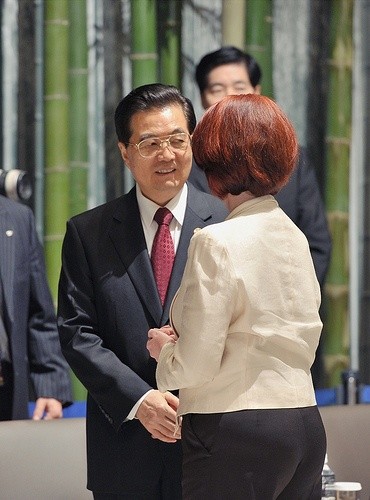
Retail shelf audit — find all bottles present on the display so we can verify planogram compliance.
[321,453,335,497]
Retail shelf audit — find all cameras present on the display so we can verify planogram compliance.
[0,168,33,201]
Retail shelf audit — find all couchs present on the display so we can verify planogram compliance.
[0,404,370,500]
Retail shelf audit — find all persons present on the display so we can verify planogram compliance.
[189,44,334,391]
[56,83,230,500]
[0,198,75,420]
[146,94,327,500]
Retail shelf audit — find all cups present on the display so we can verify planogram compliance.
[334,482,362,500]
[342,371,359,405]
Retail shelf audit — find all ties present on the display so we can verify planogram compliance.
[151,209,174,308]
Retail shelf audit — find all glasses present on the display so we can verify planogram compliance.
[123,132,190,156]
